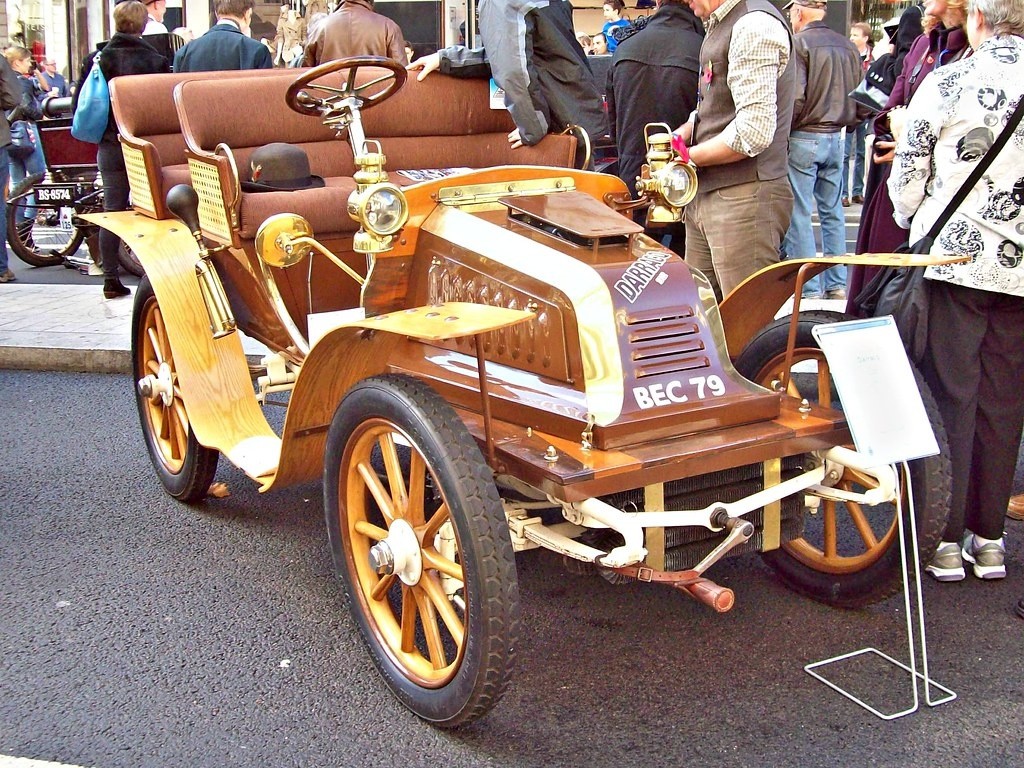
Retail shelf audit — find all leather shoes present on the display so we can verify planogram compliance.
[852,195,865,204]
[841,197,850,207]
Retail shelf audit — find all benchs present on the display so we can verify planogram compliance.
[107,65,593,250]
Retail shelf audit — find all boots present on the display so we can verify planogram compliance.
[13,217,41,252]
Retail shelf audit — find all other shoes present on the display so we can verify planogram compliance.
[806,295,820,299]
[820,289,847,301]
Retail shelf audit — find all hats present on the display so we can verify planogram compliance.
[240,143,325,191]
[782,0,827,10]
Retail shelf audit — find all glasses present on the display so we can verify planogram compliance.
[786,9,798,19]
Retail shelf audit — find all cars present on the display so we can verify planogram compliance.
[74,54,974,728]
[5,95,148,279]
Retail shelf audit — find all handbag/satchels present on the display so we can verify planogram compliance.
[8,120,35,159]
[853,237,935,364]
[848,6,925,113]
[71,51,109,144]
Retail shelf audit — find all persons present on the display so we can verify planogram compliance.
[0,45,70,284]
[171,0,273,73]
[274,0,338,66]
[404,0,1024,582]
[70,0,171,299]
[288,0,414,68]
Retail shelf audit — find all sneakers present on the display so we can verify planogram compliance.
[925,544,966,582]
[104,279,130,298]
[0,269,16,282]
[962,534,1006,579]
[1005,494,1024,520]
